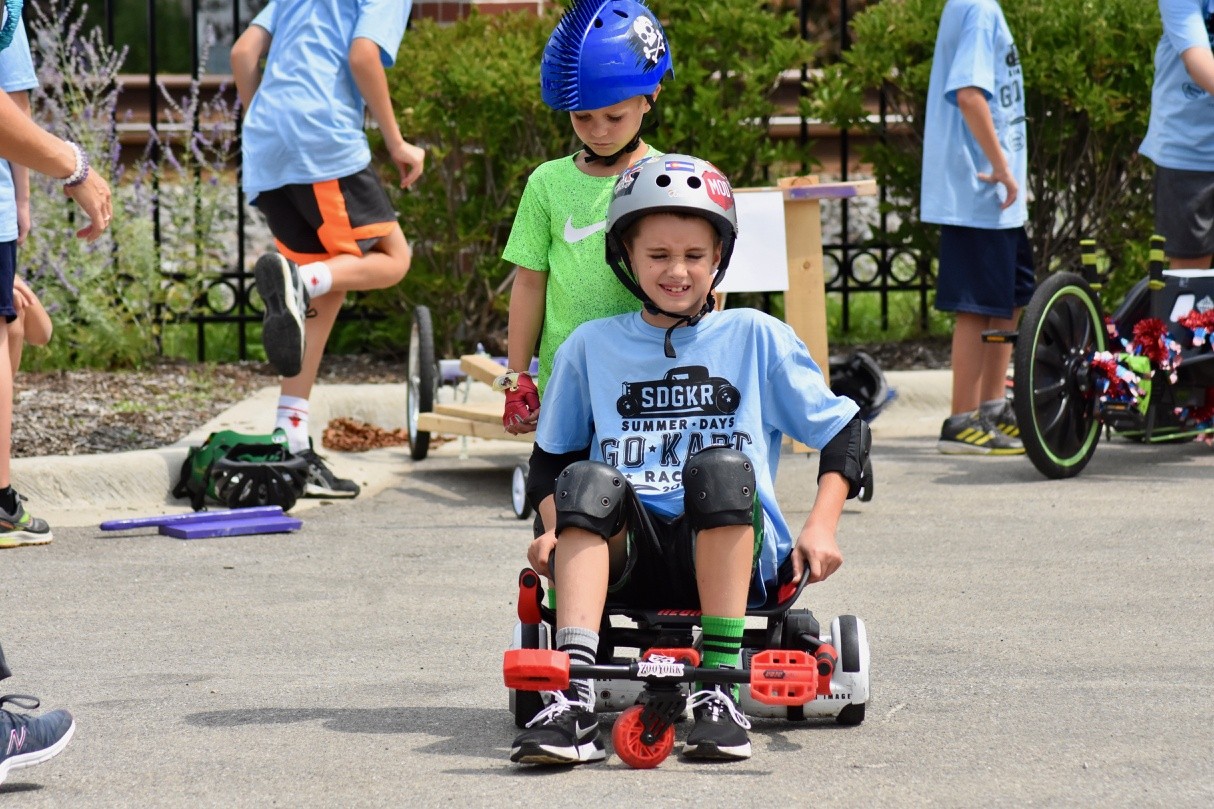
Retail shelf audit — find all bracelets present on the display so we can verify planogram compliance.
[59,141,91,188]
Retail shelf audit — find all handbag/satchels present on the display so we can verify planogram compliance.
[173,428,289,514]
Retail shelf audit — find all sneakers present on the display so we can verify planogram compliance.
[940,407,1025,457]
[0,694,75,786]
[294,436,361,499]
[981,397,1025,440]
[508,682,605,764]
[255,251,317,377]
[1,484,53,547]
[684,684,754,760]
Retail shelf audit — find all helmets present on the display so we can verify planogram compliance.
[539,0,675,111]
[604,153,739,266]
[830,348,888,427]
[208,441,308,514]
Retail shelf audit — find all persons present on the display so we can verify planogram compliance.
[1138,0,1214,269]
[160,0,425,499]
[919,1,1040,455]
[500,0,685,435]
[0,0,113,784]
[508,153,871,765]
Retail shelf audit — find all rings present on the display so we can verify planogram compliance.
[102,214,108,220]
